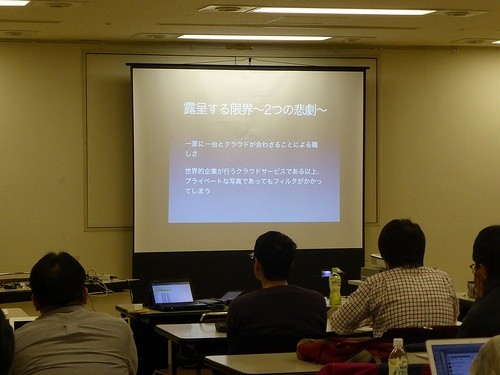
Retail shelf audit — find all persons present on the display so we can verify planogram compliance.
[0,251,138,375]
[329,219,460,338]
[457,225,500,375]
[226,231,327,355]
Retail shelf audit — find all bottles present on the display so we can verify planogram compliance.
[388,338,408,375]
[329,268,342,305]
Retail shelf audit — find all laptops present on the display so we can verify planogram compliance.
[150,282,207,310]
[424,337,492,375]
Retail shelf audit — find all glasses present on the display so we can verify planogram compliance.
[470,263,476,270]
[249,252,255,259]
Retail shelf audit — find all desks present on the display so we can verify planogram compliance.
[113,296,463,375]
